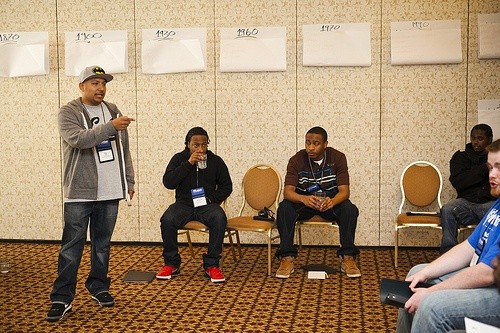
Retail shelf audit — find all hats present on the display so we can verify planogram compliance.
[79,64,113,83]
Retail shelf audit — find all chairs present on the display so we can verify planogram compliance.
[221,163,282,275]
[178,194,236,272]
[395,161,443,268]
[294,215,338,266]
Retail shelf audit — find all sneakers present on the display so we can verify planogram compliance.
[275,256,294,278]
[156,265,180,279]
[340,255,361,277]
[204,266,225,282]
[86,288,114,305]
[46,301,72,321]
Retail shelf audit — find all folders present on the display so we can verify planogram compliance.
[380,277,435,308]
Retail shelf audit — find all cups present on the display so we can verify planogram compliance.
[315,192,326,208]
[0,258,10,273]
[198,154,207,169]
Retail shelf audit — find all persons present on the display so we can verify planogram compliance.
[395,139,500,333]
[275,126,362,277]
[47,65,135,322]
[440,123,498,256]
[155,127,233,283]
